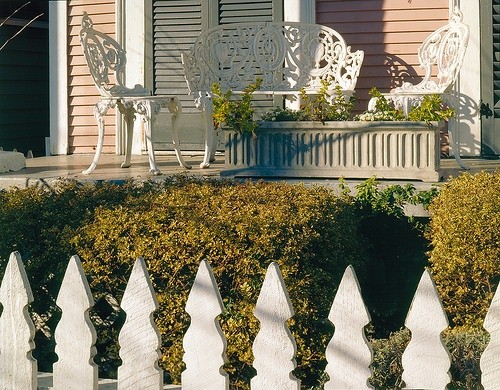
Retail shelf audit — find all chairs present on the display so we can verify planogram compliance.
[367,21,471,173]
[78,10,193,176]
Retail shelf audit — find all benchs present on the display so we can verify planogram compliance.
[180,21,366,169]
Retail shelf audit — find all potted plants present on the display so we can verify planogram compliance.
[207,77,457,183]
